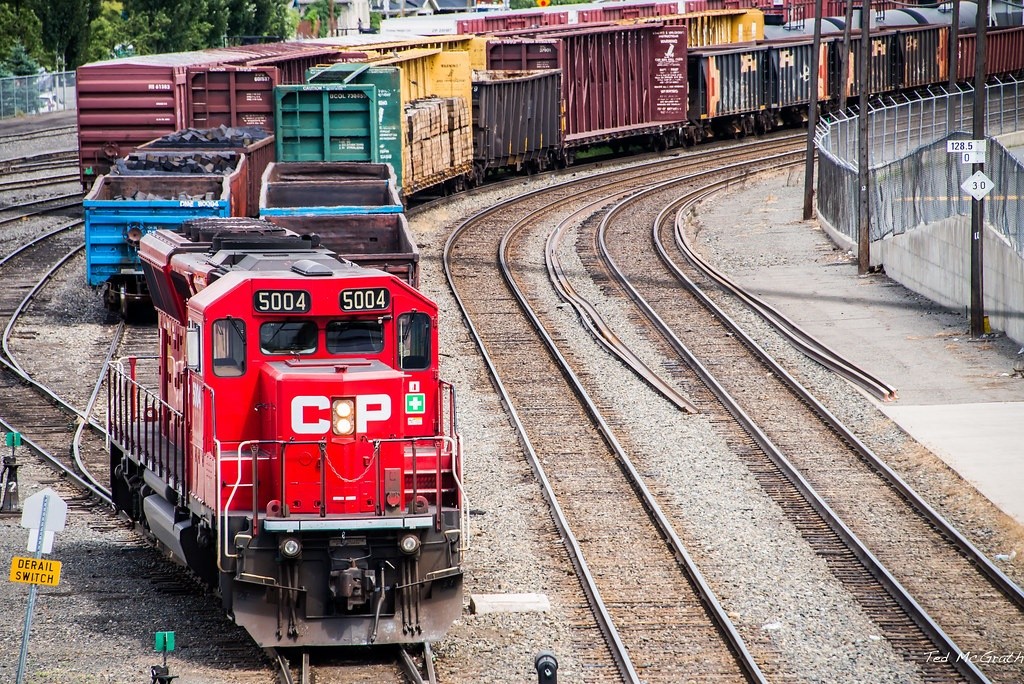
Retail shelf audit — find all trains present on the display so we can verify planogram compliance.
[77,1,1024,647]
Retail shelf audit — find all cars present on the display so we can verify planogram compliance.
[36,67,54,91]
[38,93,67,112]
